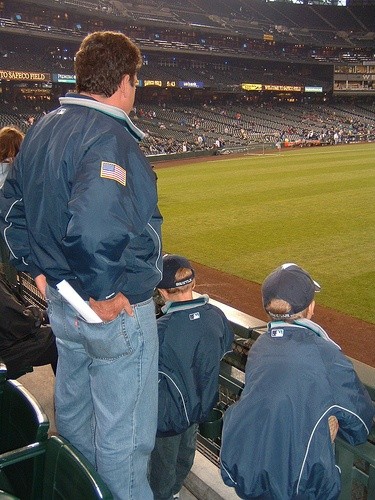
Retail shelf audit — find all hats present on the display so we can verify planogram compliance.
[261,263,322,318]
[153,255,196,291]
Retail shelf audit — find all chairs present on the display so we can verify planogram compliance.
[0,101,375,148]
[0,360,114,500]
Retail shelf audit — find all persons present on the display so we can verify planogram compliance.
[1,32,169,500]
[0,125,26,189]
[220,262,372,500]
[154,254,236,500]
[23,93,375,158]
[1,255,60,383]
